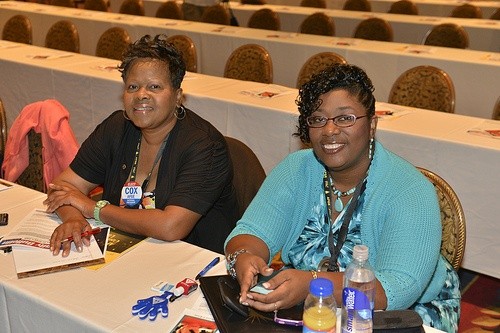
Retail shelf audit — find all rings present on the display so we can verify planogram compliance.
[273,303,278,313]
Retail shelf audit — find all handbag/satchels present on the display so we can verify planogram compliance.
[199,273,305,333]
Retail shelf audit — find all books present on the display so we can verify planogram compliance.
[12,234,106,279]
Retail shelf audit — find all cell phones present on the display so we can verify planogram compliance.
[249,270,283,295]
[274,302,306,327]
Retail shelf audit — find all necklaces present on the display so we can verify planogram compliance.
[329,171,356,213]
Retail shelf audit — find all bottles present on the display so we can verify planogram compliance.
[302,279,336,333]
[340,246,374,333]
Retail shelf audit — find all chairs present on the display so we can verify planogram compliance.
[0,99,81,194]
[416,167,466,271]
[225,135,266,219]
[81,0,500,49]
[96,27,131,60]
[389,65,456,114]
[3,14,32,45]
[168,35,197,73]
[44,20,80,53]
[223,44,273,84]
[296,52,348,89]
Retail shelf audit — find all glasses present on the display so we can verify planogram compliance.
[307,113,368,127]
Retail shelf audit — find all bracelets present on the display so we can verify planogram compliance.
[226,249,254,280]
[311,269,317,280]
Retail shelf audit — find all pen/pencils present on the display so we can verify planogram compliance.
[60,227,101,242]
[195,257,220,280]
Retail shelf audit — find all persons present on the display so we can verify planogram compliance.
[43,34,240,257]
[223,62,461,333]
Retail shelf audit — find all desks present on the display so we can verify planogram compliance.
[108,0,500,53]
[0,179,230,333]
[263,0,500,21]
[0,37,500,279]
[0,0,500,120]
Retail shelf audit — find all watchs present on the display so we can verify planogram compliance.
[94,200,110,221]
